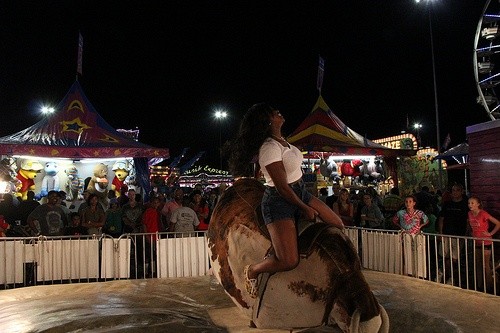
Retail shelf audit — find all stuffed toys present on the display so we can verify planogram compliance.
[314,154,385,180]
[33,159,61,200]
[86,162,109,199]
[11,160,44,200]
[63,165,85,201]
[110,160,132,199]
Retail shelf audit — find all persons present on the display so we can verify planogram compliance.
[358,192,383,229]
[335,175,464,203]
[464,195,500,291]
[327,183,341,211]
[381,187,404,230]
[332,188,355,227]
[166,196,200,237]
[440,187,452,211]
[413,185,436,212]
[78,193,106,240]
[25,219,41,237]
[420,204,438,277]
[433,183,471,284]
[243,101,345,299]
[392,194,430,232]
[140,197,163,278]
[0,213,10,237]
[26,190,68,240]
[186,189,210,232]
[120,188,143,279]
[318,187,329,203]
[103,199,124,238]
[0,175,232,227]
[59,212,89,240]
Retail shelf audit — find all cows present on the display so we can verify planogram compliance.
[206,144,389,333]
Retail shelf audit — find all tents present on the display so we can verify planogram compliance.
[0,34,170,197]
[249,54,418,190]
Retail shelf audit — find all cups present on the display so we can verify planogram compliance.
[361,214,366,226]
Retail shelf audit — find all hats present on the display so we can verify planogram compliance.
[109,198,119,204]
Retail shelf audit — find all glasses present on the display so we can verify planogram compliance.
[452,189,460,192]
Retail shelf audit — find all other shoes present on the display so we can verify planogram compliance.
[244,264,259,298]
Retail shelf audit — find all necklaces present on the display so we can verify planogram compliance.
[271,133,286,142]
